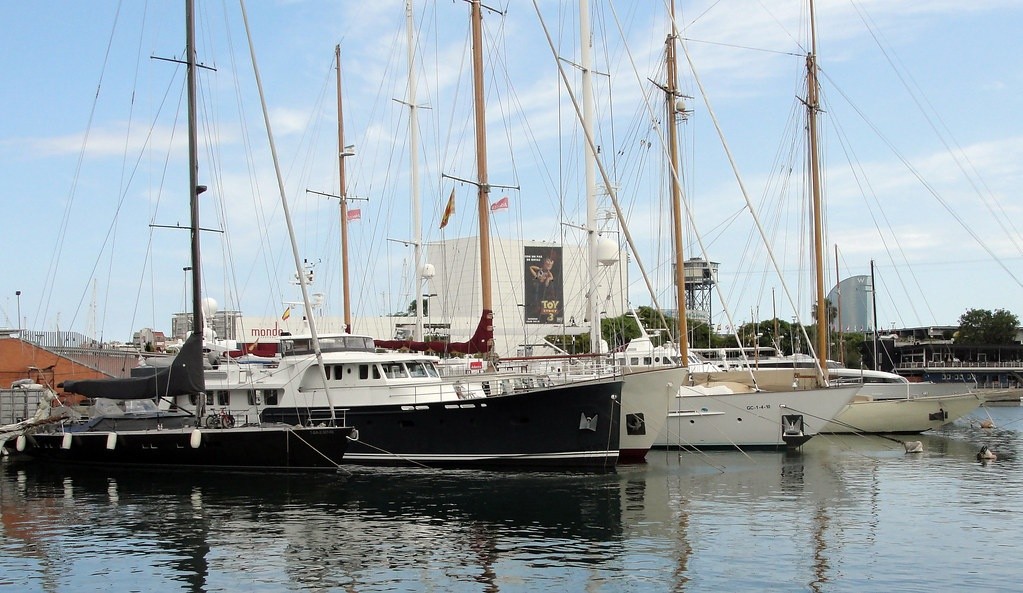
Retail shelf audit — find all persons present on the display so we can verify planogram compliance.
[454,380,465,399]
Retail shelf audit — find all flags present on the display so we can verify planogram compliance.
[282,307,290,321]
[249,338,259,351]
[439,188,456,229]
[347,209,361,223]
[490,197,508,212]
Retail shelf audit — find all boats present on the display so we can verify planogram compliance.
[124,1,689,473]
[0,1,351,465]
[663,0,989,450]
